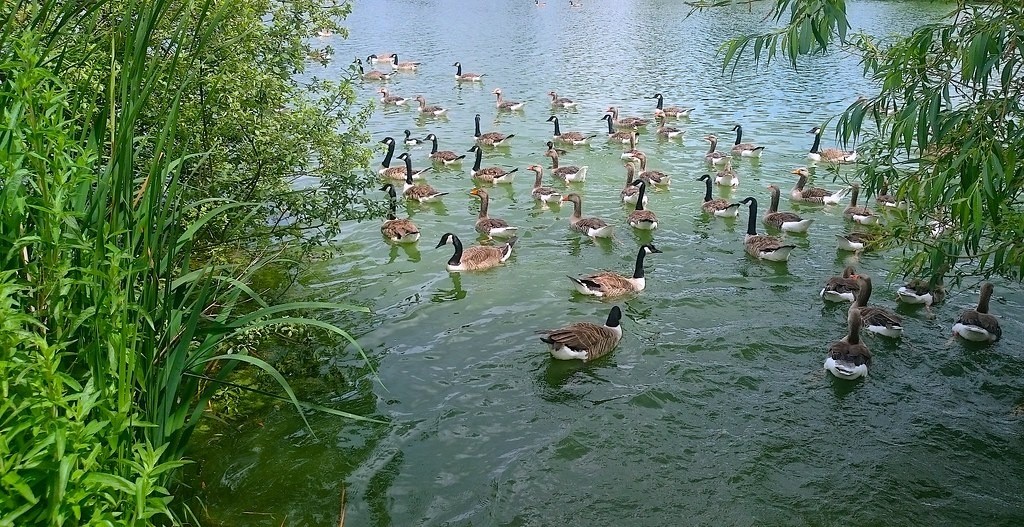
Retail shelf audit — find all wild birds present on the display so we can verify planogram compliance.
[568,1,583,7]
[540,305,623,361]
[403,130,423,145]
[897,263,947,307]
[929,207,955,240]
[414,95,448,115]
[652,93,696,117]
[470,189,518,240]
[467,146,519,184]
[377,87,413,105]
[546,116,597,146]
[388,54,421,70]
[695,174,742,218]
[620,161,648,206]
[395,153,450,203]
[526,165,564,204]
[543,141,568,156]
[714,155,740,187]
[738,196,797,262]
[842,183,888,226]
[535,0,546,7]
[561,193,615,239]
[601,114,639,145]
[805,126,857,164]
[473,114,516,147]
[352,59,390,80]
[761,184,816,233]
[452,61,485,81]
[423,134,466,166]
[631,151,672,186]
[376,137,435,180]
[730,124,766,158]
[565,243,663,297]
[820,266,861,302]
[542,149,589,185]
[823,302,876,381]
[365,54,395,62]
[378,184,421,243]
[789,167,853,205]
[952,282,1003,345]
[653,110,686,138]
[626,179,659,231]
[848,273,904,338]
[606,106,650,126]
[834,232,876,254]
[621,130,639,161]
[434,233,519,274]
[876,176,917,212]
[317,28,332,37]
[856,95,900,116]
[548,91,578,108]
[491,88,523,111]
[703,134,730,165]
